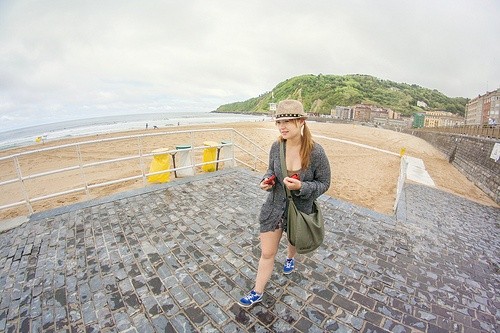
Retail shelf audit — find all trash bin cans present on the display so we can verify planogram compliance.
[176,146,194,177]
[202,141,218,172]
[222,141,233,168]
[148,148,170,183]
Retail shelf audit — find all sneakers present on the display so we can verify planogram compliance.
[282,255,294,274]
[238,290,263,307]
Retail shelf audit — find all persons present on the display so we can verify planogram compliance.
[237,100,331,308]
[153,125,158,130]
[145,122,148,130]
[489,118,496,129]
[177,121,179,126]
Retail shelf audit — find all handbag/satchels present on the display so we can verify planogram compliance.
[287,199,324,254]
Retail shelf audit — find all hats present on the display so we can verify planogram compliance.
[274,100,308,121]
